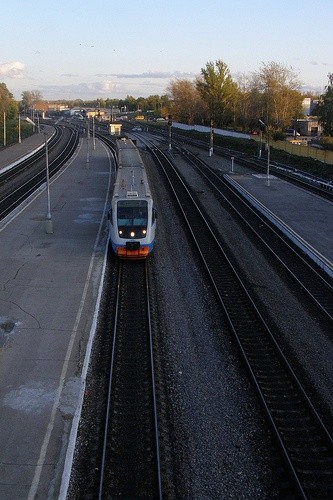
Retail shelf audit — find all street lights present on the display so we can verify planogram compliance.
[91,114,96,150]
[25,117,53,219]
[258,119,271,187]
[78,114,90,162]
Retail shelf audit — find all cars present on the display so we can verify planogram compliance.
[284,129,300,136]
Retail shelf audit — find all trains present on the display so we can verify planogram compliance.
[108,138,156,260]
